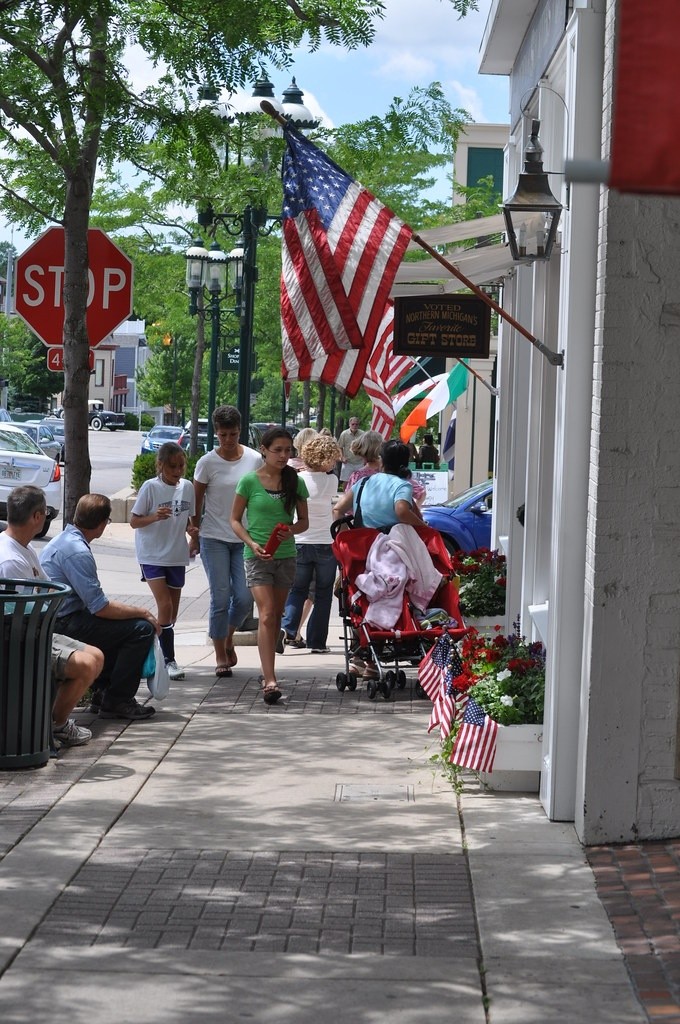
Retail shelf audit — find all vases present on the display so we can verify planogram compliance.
[481,724,542,770]
[463,615,504,636]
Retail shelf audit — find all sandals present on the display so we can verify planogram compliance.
[349,663,367,677]
[362,669,382,681]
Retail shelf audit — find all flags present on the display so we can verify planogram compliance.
[362,298,416,441]
[450,696,498,773]
[280,122,413,399]
[417,630,470,741]
[391,358,470,445]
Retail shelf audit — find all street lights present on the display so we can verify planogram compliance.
[184,237,244,450]
[196,75,318,453]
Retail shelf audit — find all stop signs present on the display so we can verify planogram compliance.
[14,226,132,349]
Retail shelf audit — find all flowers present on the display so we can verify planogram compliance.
[430,624,545,795]
[449,546,507,617]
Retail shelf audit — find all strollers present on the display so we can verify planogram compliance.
[329,515,469,700]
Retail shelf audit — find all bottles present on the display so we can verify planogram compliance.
[262,523,288,559]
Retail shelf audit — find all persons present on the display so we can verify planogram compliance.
[38,493,162,720]
[333,439,427,680]
[286,428,321,648]
[275,436,343,654]
[320,428,332,436]
[337,417,366,492]
[130,442,199,679]
[0,485,104,751]
[230,427,310,705]
[189,405,266,676]
[345,430,427,515]
[407,433,440,469]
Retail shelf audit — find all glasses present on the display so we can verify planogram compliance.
[104,515,112,525]
[35,508,51,515]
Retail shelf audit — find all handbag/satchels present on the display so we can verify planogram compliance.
[141,633,169,700]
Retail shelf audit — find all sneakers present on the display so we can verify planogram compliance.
[52,718,92,745]
[166,659,186,680]
[98,697,156,719]
[90,693,101,713]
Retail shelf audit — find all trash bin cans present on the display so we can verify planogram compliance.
[0,577,74,771]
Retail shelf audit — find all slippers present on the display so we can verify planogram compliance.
[263,685,281,702]
[225,646,237,667]
[214,662,232,676]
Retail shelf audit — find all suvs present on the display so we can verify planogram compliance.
[178,419,220,452]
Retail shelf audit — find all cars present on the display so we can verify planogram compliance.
[0,408,13,422]
[141,424,183,455]
[418,476,492,555]
[38,419,66,454]
[88,400,125,432]
[248,422,299,456]
[0,422,63,465]
[0,424,62,519]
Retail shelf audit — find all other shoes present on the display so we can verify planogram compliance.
[312,645,331,654]
[288,634,307,648]
[275,628,287,655]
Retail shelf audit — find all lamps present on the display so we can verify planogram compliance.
[497,118,570,264]
